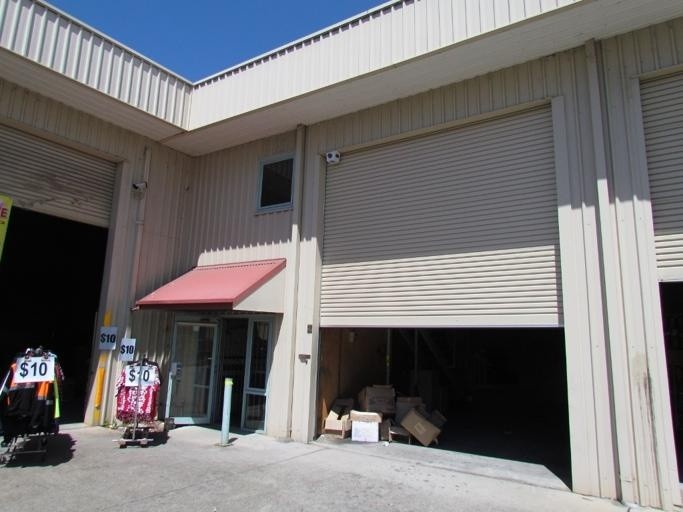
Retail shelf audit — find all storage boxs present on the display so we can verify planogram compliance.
[318,377,449,447]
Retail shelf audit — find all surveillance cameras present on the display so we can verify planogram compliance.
[132,181,147,192]
